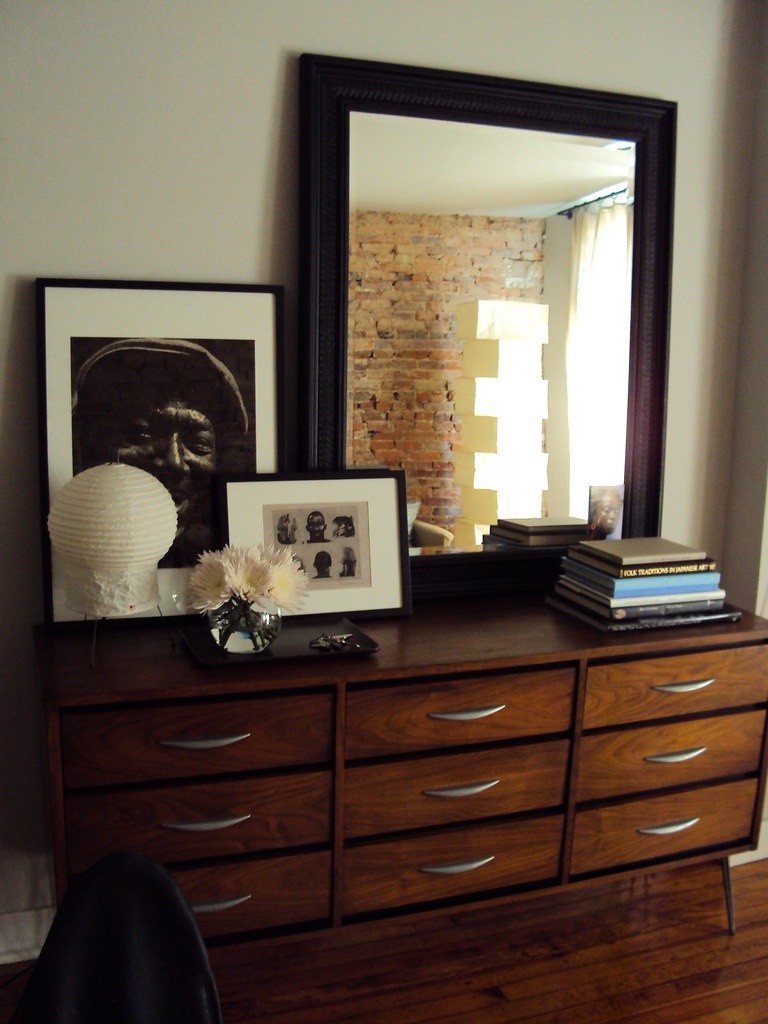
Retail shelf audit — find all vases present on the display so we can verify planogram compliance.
[207,598,281,654]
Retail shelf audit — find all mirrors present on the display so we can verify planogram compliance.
[298,50,676,605]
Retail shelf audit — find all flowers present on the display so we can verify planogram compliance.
[182,542,310,614]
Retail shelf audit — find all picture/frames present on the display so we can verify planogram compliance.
[34,277,289,633]
[212,469,414,626]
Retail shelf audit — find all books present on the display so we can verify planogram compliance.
[482,516,589,553]
[544,536,743,632]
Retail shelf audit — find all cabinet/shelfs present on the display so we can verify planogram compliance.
[33,597,768,936]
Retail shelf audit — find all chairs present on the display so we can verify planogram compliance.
[0,849,223,1024]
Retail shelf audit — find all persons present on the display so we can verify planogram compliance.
[306,511,329,543]
[313,551,331,578]
[72,338,248,567]
[590,487,624,539]
